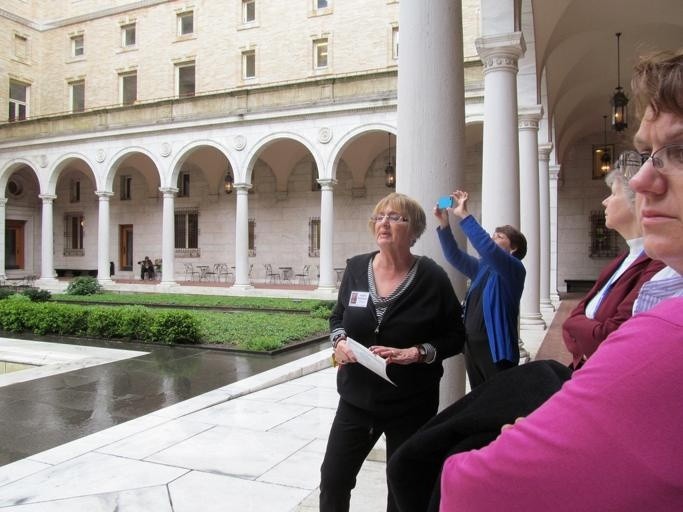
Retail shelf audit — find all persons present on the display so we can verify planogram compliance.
[140,256,154,281]
[433,188,528,391]
[561,162,665,371]
[439,50,682,510]
[319,193,466,512]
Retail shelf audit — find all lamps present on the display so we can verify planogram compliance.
[385,133,395,187]
[224,163,233,194]
[600,116,611,177]
[610,31,630,132]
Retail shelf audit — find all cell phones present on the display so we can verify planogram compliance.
[439,196,453,209]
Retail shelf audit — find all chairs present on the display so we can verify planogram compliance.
[183,261,320,289]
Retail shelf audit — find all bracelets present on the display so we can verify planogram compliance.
[330,334,347,347]
[332,337,347,349]
[412,344,426,363]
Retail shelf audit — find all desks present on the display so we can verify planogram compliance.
[333,268,345,284]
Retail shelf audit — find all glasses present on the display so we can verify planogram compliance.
[617,143,683,179]
[370,215,410,223]
